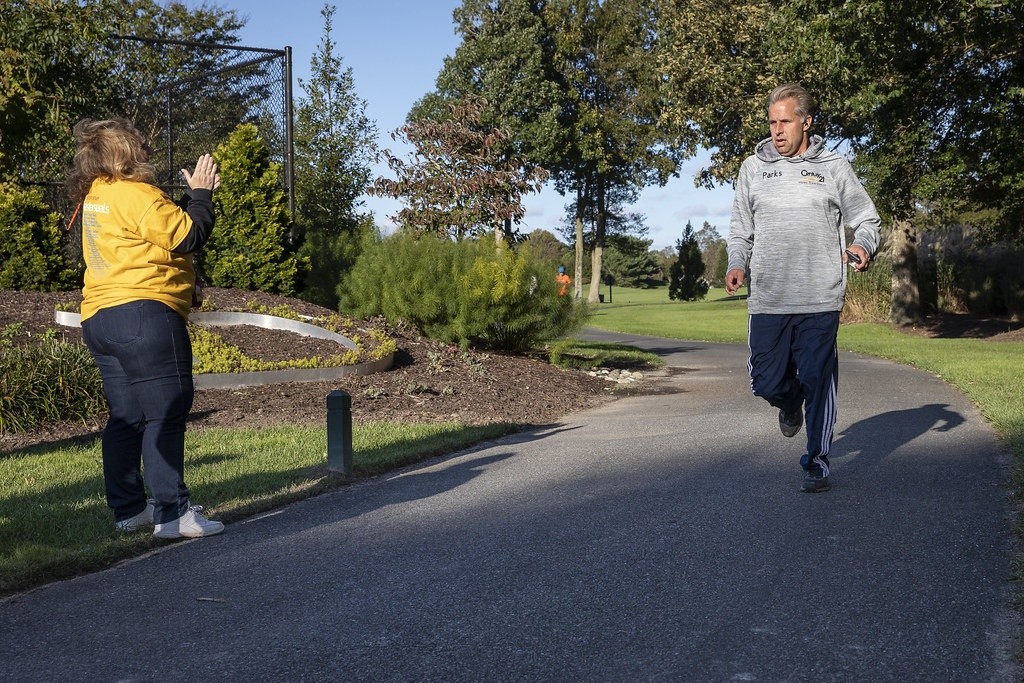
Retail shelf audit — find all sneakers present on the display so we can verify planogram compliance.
[779,407,803,438]
[801,473,830,492]
[117,498,156,530]
[152,500,225,537]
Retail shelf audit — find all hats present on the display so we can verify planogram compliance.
[558,266,565,273]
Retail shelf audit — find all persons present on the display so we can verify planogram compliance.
[72,118,225,540]
[553,266,571,296]
[724,81,883,494]
